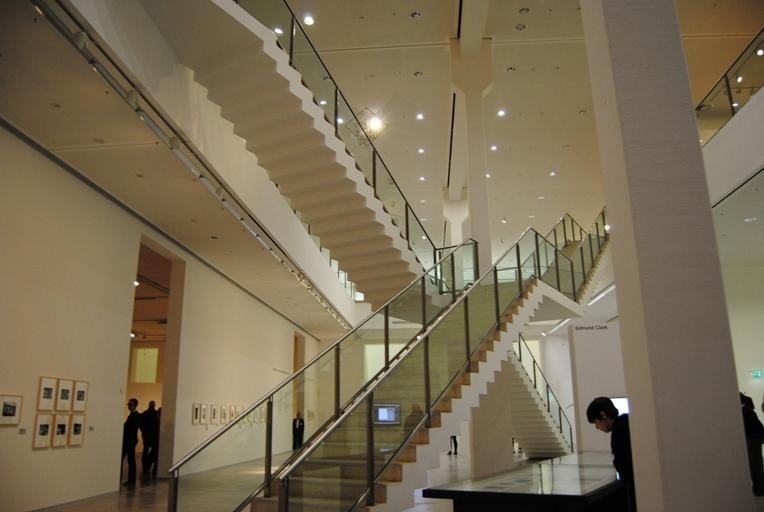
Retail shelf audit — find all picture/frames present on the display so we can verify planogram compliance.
[1,394,24,426]
[192,404,253,422]
[32,377,89,449]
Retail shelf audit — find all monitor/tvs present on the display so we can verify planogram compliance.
[371,404,401,426]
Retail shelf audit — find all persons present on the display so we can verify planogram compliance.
[120,398,140,490]
[292,412,303,450]
[738,391,764,496]
[402,403,424,441]
[585,396,637,511]
[138,400,160,482]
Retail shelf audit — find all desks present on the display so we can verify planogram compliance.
[422,450,622,512]
